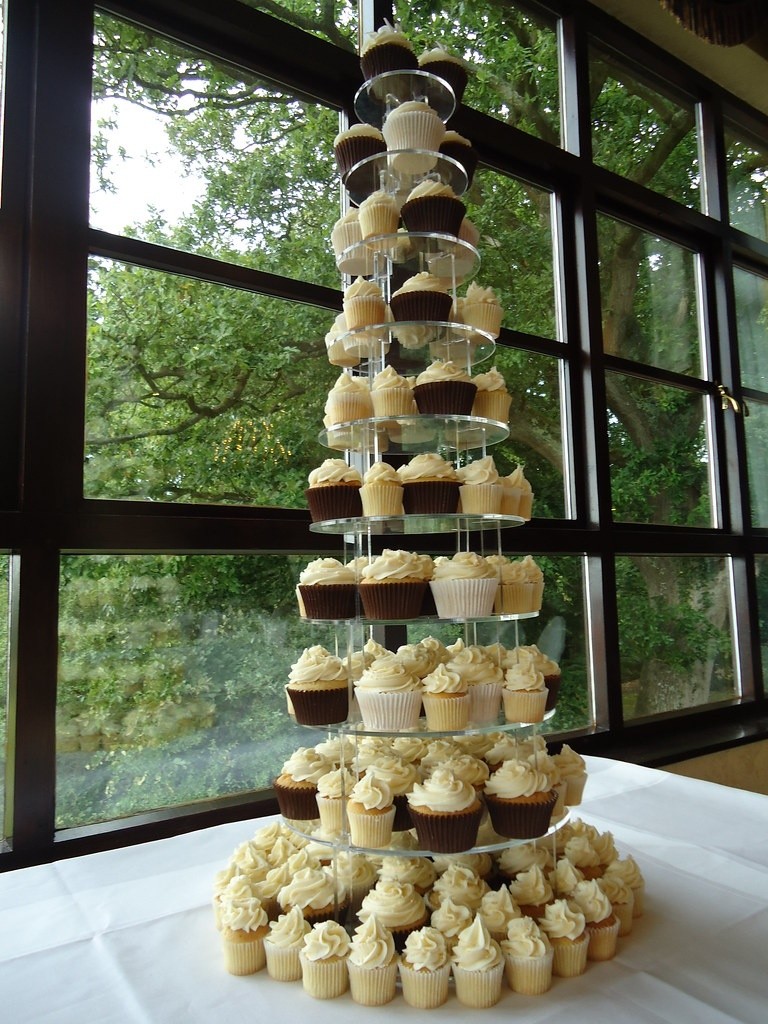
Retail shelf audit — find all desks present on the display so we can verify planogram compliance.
[1,755,768,1024]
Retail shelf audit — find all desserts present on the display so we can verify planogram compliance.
[219,26,645,1005]
